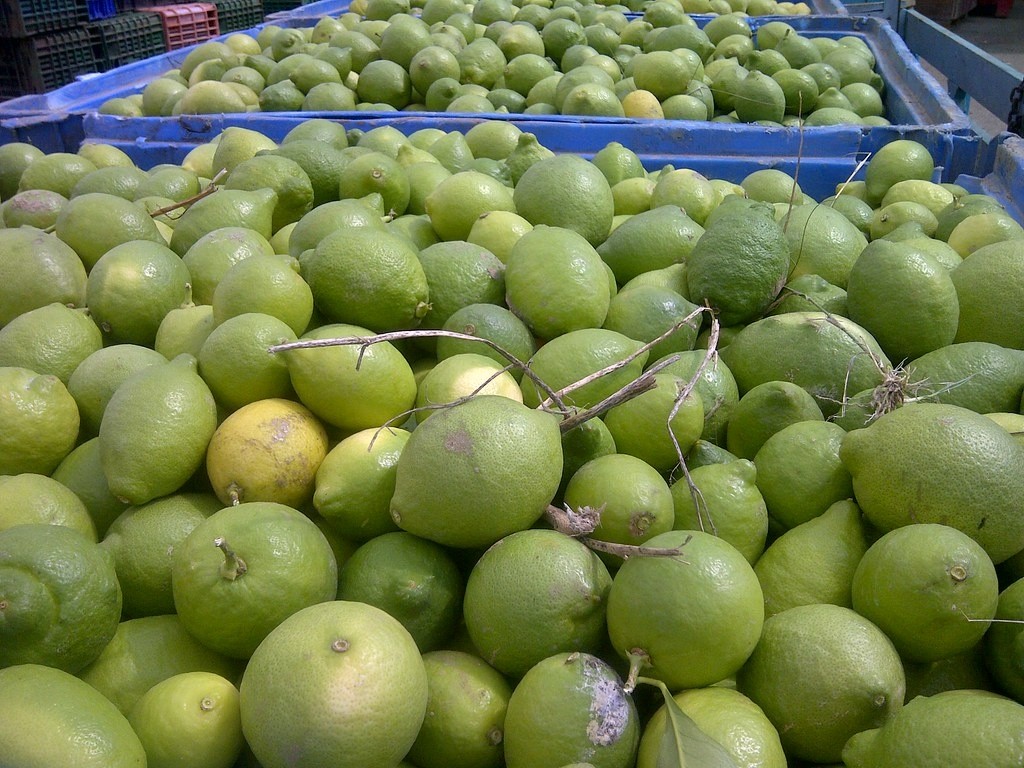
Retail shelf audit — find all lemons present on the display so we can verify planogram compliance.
[0,118,1024,768]
[95,0,897,129]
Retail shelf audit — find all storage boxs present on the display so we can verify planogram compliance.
[0,0,1024,225]
